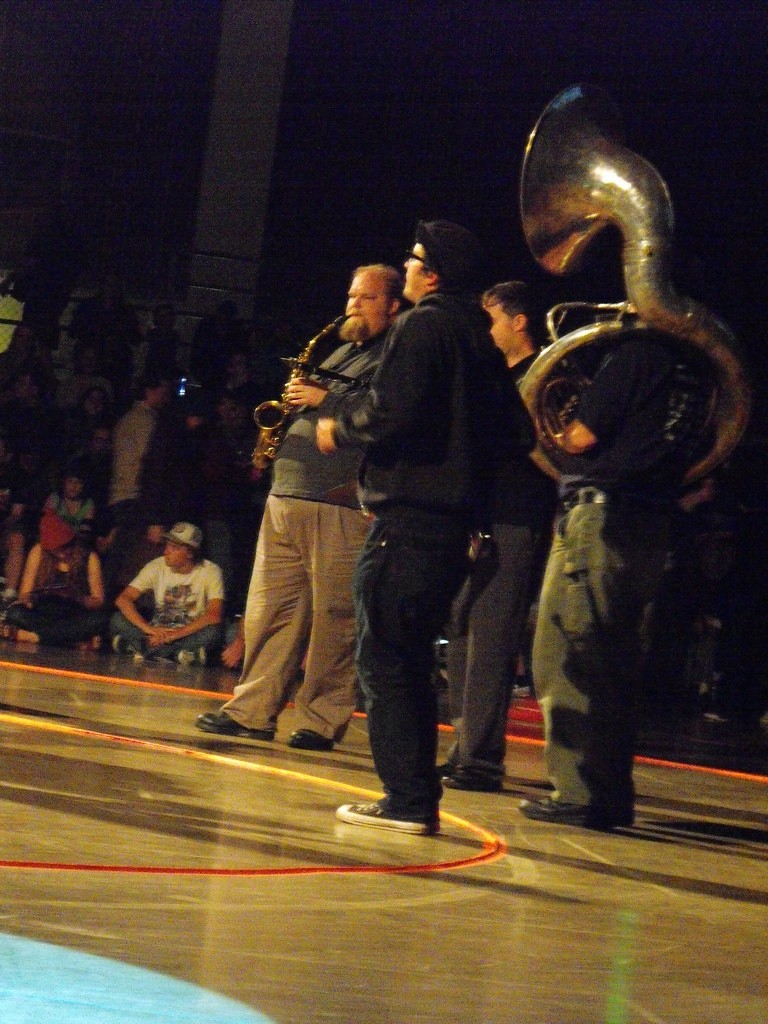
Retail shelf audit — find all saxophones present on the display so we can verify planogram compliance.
[247,314,359,478]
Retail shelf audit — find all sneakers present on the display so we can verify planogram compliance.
[335,803,442,836]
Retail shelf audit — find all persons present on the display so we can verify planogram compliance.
[196,265,411,752]
[0,304,768,730]
[285,220,535,836]
[520,339,709,829]
[435,281,555,793]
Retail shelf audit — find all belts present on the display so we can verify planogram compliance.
[560,491,603,515]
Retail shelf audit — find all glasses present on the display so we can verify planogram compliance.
[399,244,434,271]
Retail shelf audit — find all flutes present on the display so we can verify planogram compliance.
[280,357,368,389]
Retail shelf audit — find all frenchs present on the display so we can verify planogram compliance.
[510,83,755,513]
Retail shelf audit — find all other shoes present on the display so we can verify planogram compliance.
[435,760,505,793]
[109,633,137,655]
[519,793,638,830]
[1,622,25,641]
[176,649,195,666]
[86,634,102,651]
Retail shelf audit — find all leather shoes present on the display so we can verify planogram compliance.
[194,712,276,741]
[288,729,336,749]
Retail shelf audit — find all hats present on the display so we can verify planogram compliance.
[38,508,76,551]
[158,520,203,549]
